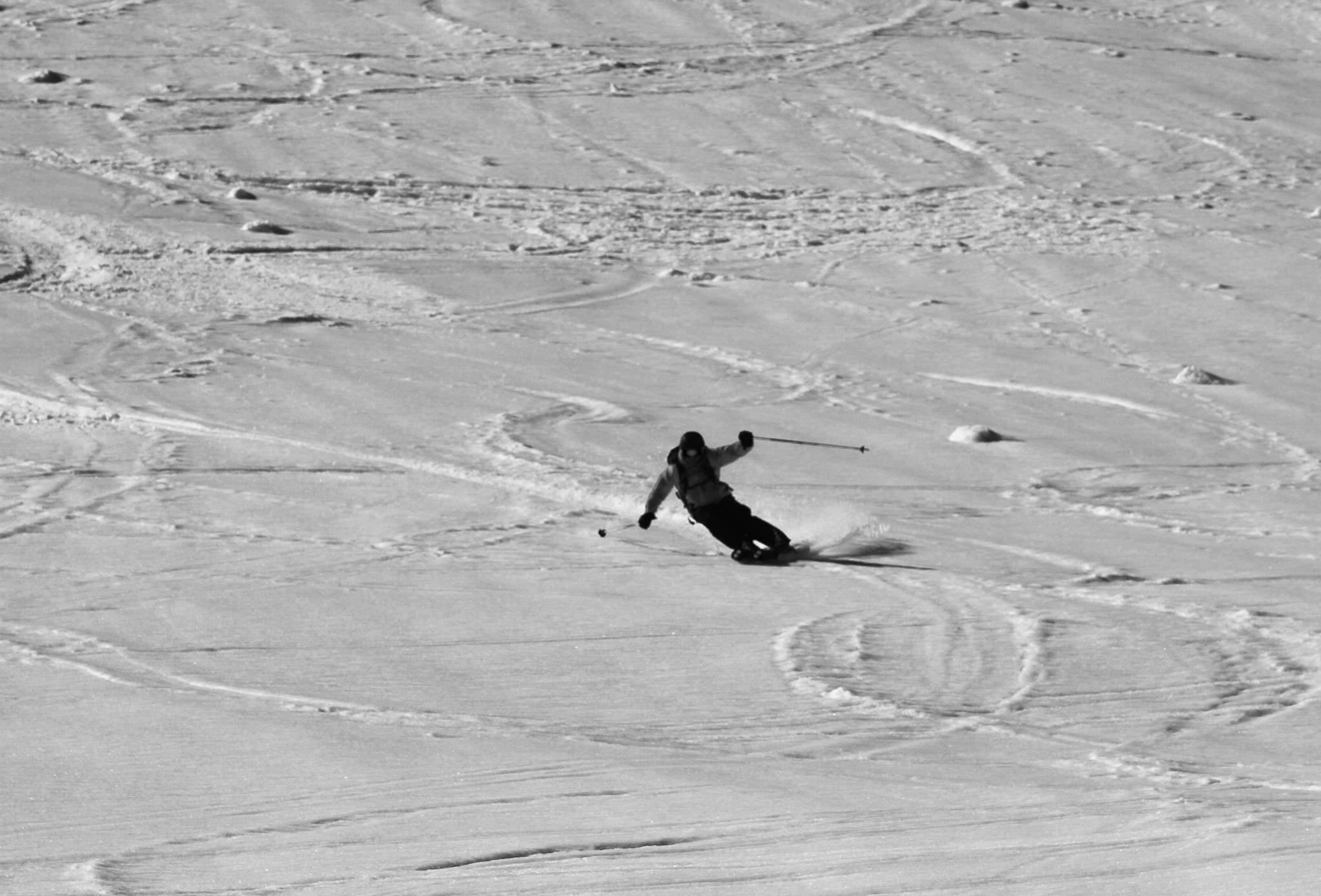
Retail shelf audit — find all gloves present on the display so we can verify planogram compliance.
[738,430,754,447]
[639,512,655,529]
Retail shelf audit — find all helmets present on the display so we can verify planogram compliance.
[681,431,704,449]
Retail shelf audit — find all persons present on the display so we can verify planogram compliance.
[638,430,798,563]
